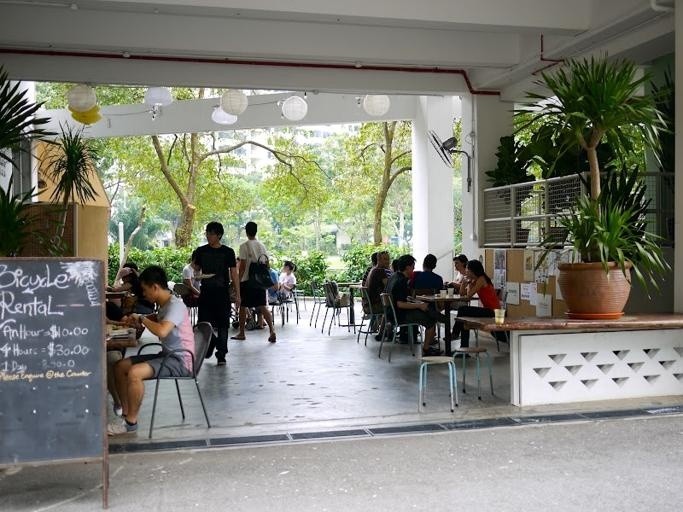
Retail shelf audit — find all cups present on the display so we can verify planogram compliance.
[439,290,446,298]
[447,288,454,296]
[494,308,506,324]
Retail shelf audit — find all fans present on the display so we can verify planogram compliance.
[427,130,471,193]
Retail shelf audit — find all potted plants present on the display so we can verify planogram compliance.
[535,196,670,319]
[486,128,615,249]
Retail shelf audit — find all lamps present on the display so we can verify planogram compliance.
[220,88,249,114]
[64,82,97,112]
[363,94,392,116]
[212,108,238,125]
[276,94,308,122]
[69,103,101,125]
[144,85,175,107]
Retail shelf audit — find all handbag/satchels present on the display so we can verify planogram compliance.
[324,280,352,307]
[249,253,275,288]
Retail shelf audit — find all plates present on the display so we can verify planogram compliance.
[104,324,132,341]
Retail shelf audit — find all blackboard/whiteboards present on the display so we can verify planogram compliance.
[0,257,106,468]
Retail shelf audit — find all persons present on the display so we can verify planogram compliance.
[193,222,241,366]
[443,259,501,358]
[106,261,296,330]
[106,299,125,417]
[444,255,468,295]
[361,251,444,357]
[230,221,276,343]
[106,265,196,435]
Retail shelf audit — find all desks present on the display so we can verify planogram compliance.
[337,281,367,327]
[417,294,468,355]
[454,315,683,331]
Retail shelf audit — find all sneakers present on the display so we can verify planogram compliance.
[107,404,137,436]
[218,357,226,365]
[424,346,445,356]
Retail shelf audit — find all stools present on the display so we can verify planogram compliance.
[453,347,496,399]
[418,354,459,414]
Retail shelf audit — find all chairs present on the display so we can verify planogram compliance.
[172,281,309,327]
[137,321,214,440]
[309,281,358,336]
[378,291,430,363]
[358,286,384,348]
[467,285,507,353]
